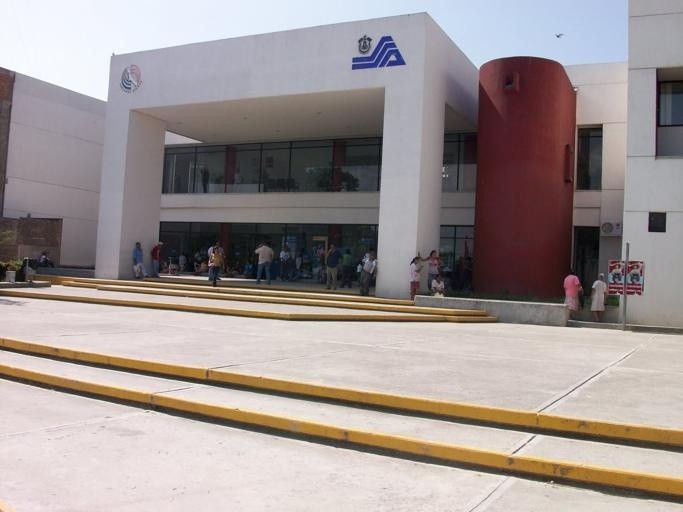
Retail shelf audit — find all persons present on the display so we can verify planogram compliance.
[151,242,163,277]
[40,250,52,266]
[563,268,583,318]
[133,242,147,278]
[162,240,376,296]
[589,273,606,320]
[409,251,474,299]
